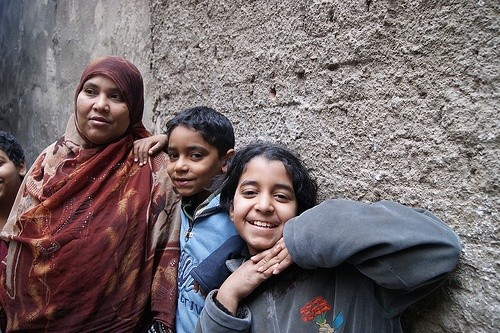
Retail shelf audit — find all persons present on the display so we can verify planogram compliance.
[1,54,181,333]
[192,142,461,333]
[0,129,27,332]
[133,105,251,332]
